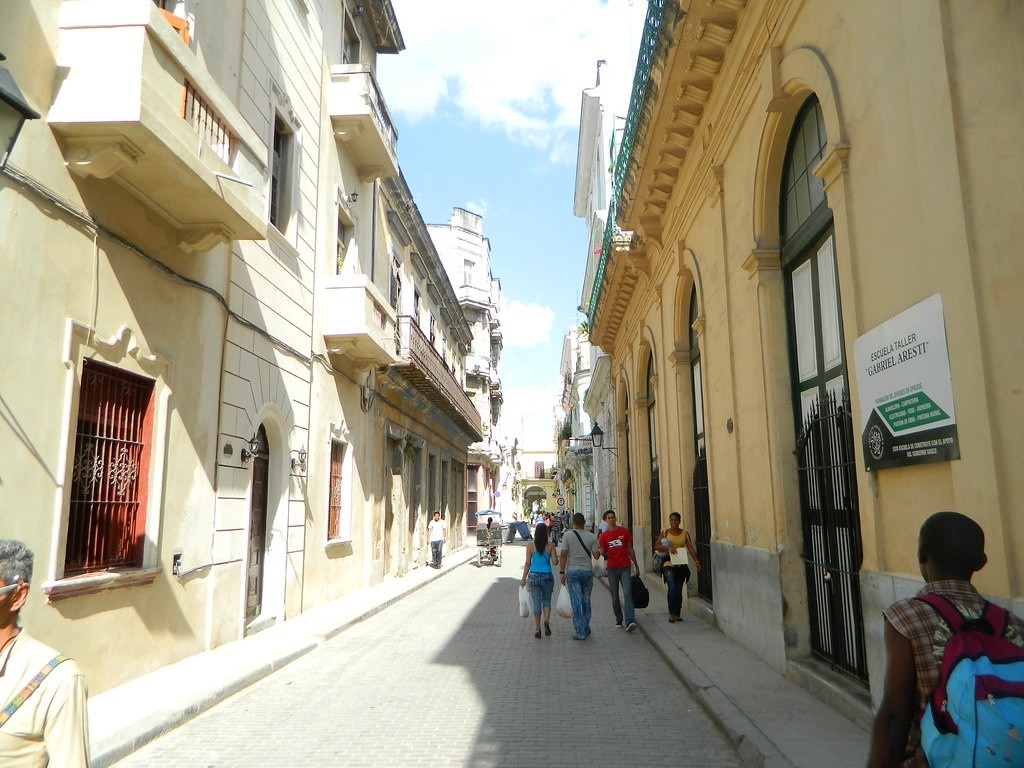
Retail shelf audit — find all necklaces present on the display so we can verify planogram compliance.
[0,635,17,654]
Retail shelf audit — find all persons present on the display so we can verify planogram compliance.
[426,511,447,569]
[597,510,640,632]
[511,513,517,534]
[521,523,559,638]
[864,512,1024,768]
[0,539,91,768]
[559,512,600,641]
[654,512,700,623]
[487,517,494,529]
[530,510,569,537]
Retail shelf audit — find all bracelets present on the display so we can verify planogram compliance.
[694,561,699,565]
[444,538,446,539]
[560,568,565,574]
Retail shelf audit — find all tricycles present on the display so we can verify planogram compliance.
[474,510,504,567]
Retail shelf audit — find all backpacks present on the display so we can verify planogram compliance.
[914,593,1024,768]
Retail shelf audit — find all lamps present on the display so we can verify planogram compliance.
[292,446,307,470]
[591,422,619,459]
[348,189,358,205]
[352,4,363,18]
[241,432,260,461]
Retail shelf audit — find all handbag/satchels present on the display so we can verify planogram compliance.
[630,574,649,608]
[518,584,530,618]
[555,583,574,618]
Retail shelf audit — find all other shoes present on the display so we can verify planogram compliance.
[535,631,541,639]
[676,611,683,621]
[626,622,636,631]
[544,620,551,637]
[615,620,622,627]
[573,636,578,639]
[669,612,675,623]
